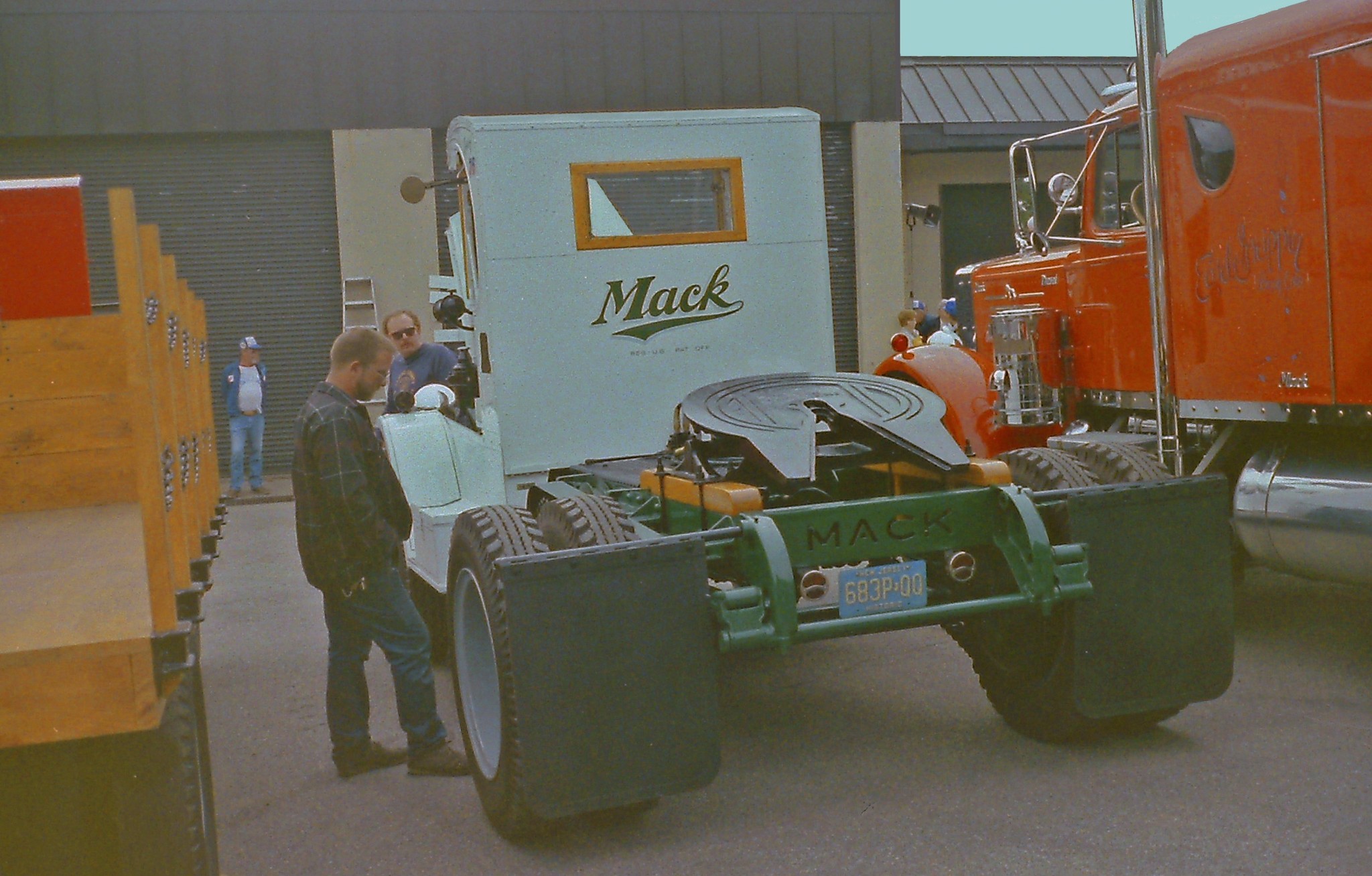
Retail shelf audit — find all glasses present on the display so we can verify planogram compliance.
[393,326,415,340]
[361,362,391,381]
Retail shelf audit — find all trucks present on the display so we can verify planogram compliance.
[869,1,1372,610]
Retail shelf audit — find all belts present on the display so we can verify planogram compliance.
[239,411,258,416]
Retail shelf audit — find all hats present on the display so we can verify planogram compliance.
[943,299,965,318]
[238,337,264,350]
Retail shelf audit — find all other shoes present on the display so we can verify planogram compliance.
[226,490,241,498]
[253,487,271,494]
[406,735,474,775]
[333,742,405,778]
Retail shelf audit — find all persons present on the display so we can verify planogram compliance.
[923,303,935,321]
[897,309,921,348]
[294,327,475,777]
[913,301,929,336]
[930,299,947,335]
[943,297,958,335]
[223,333,274,499]
[376,310,459,455]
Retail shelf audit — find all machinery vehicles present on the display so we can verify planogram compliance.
[369,105,1249,844]
